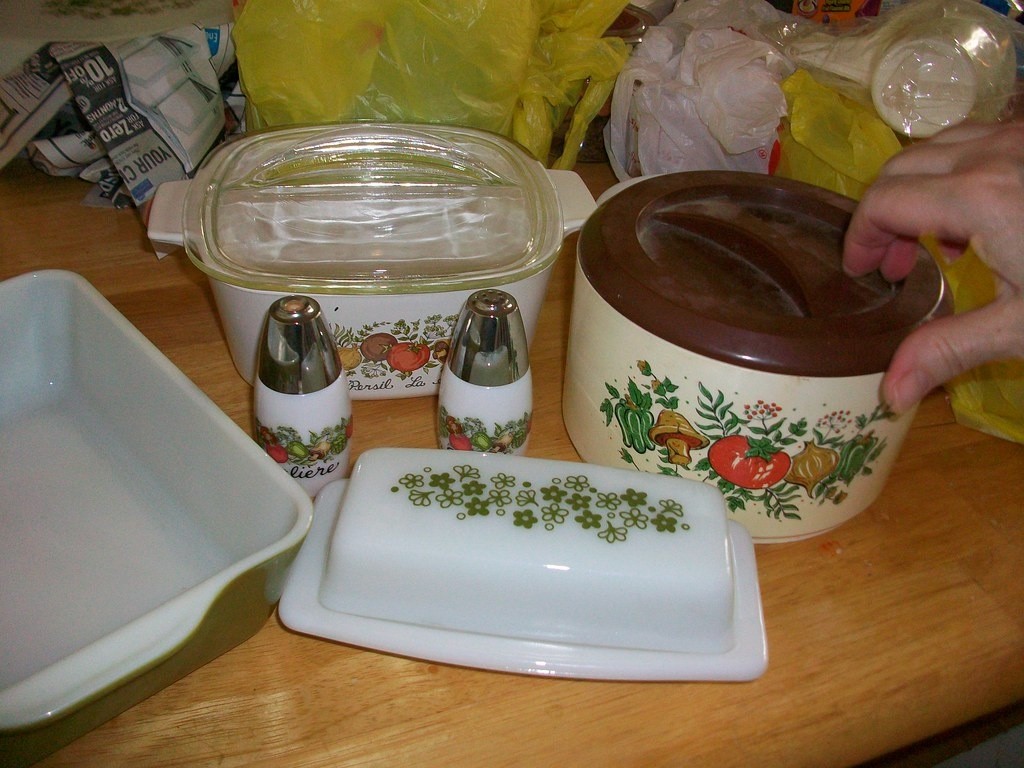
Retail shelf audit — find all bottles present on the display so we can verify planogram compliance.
[436,288,534,457]
[253,294,353,497]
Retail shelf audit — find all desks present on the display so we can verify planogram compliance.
[0,157,1022,768]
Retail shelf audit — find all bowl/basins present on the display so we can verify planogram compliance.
[277,446,771,684]
[558,168,952,545]
[0,268,316,731]
[145,118,595,403]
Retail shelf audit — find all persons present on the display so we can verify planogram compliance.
[842,121,1024,415]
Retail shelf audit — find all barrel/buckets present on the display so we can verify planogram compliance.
[871,1,1018,140]
